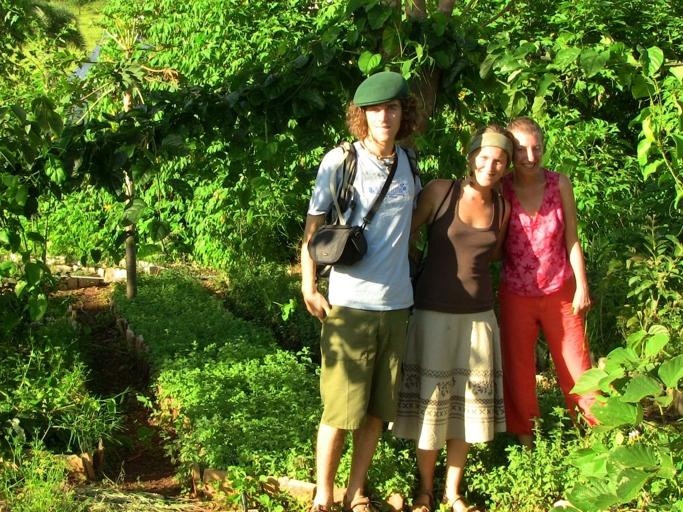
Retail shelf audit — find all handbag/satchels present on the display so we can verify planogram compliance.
[306,220,372,267]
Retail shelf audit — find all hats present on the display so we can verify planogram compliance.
[353,71,409,107]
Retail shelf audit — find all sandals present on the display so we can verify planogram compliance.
[442,495,479,512]
[411,490,435,512]
[342,495,379,512]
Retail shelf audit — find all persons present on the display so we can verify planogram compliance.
[299,70,424,511]
[494,116,610,450]
[389,125,514,512]
